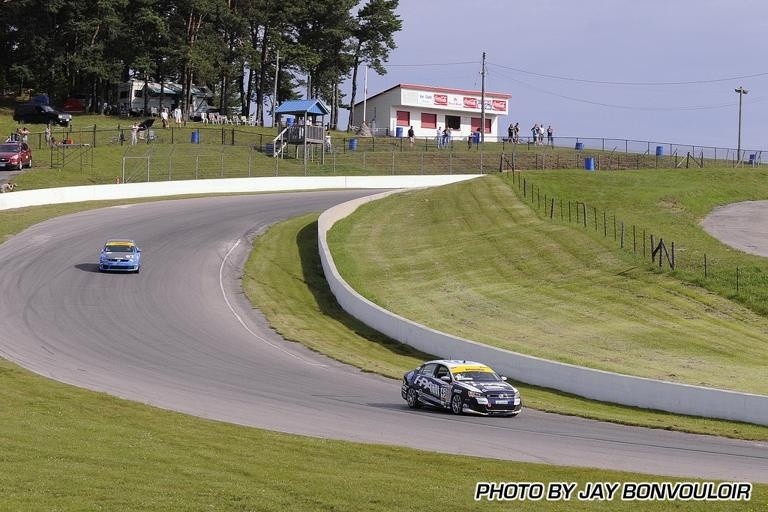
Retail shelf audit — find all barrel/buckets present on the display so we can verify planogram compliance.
[575,143,584,149]
[396,127,403,137]
[584,157,595,171]
[286,117,294,126]
[266,143,273,155]
[472,132,479,143]
[656,146,663,156]
[750,154,756,164]
[191,130,200,144]
[349,138,359,150]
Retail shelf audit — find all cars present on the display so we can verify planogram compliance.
[0,141,32,170]
[14,103,72,125]
[63,98,85,114]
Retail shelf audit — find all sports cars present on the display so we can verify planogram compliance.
[402,354,522,417]
[99,238,142,272]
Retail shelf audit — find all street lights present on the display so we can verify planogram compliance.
[734,86,751,161]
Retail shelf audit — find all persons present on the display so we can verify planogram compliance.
[507,123,514,143]
[298,116,305,126]
[442,125,452,146]
[466,132,476,152]
[45,122,52,143]
[22,127,30,145]
[408,125,416,149]
[324,131,332,153]
[174,104,183,128]
[436,126,442,149]
[514,122,521,144]
[15,127,22,143]
[130,122,144,146]
[160,108,169,127]
[531,123,554,145]
